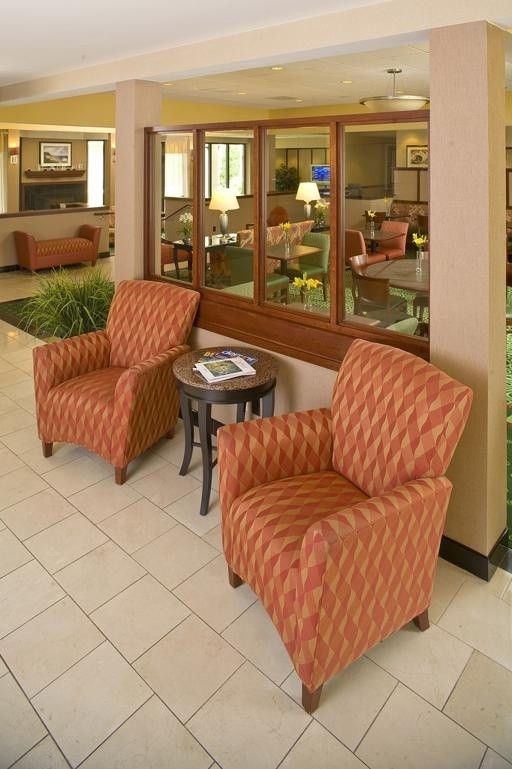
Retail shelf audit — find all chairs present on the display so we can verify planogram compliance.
[223,243,289,303]
[210,334,479,716]
[211,219,317,275]
[343,228,386,271]
[25,276,204,490]
[247,204,289,230]
[378,219,409,262]
[346,252,430,336]
[268,230,331,305]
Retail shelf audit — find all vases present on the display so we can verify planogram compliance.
[279,236,291,256]
[367,220,377,233]
[182,229,191,245]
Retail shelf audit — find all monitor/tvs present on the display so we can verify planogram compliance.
[311,164,331,189]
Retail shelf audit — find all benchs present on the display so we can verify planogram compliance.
[14,219,105,274]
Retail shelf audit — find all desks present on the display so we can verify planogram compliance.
[358,229,408,254]
[171,339,278,514]
[262,242,324,307]
[170,231,241,277]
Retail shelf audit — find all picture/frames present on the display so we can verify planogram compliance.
[37,141,75,167]
[404,145,429,170]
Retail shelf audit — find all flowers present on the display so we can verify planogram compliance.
[313,199,329,218]
[273,219,295,240]
[362,208,379,220]
[178,211,194,237]
[411,229,429,250]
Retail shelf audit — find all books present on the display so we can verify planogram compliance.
[197,348,259,366]
[194,356,257,383]
[193,368,256,386]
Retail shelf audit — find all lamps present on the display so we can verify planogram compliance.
[294,180,320,220]
[7,124,21,154]
[207,187,242,235]
[359,68,431,116]
